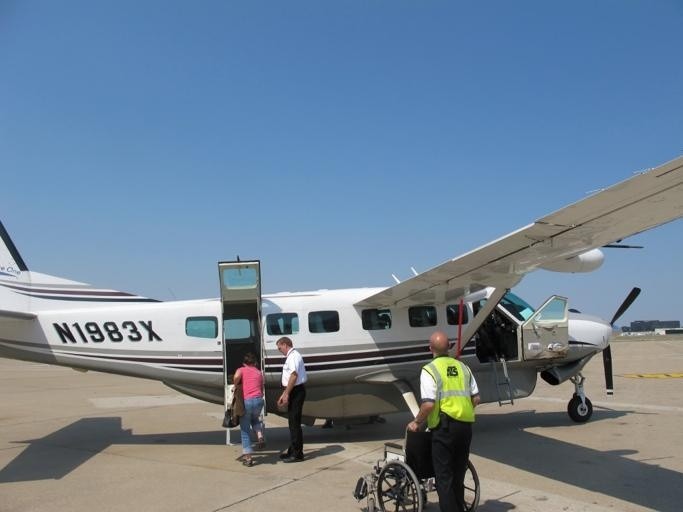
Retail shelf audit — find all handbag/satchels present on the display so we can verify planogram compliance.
[223,409,239,428]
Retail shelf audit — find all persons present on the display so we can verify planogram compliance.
[478,306,514,362]
[231,350,268,468]
[274,336,308,463]
[406,330,481,510]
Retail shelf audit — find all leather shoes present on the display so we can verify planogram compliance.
[280,454,304,463]
[259,438,264,448]
[243,460,252,467]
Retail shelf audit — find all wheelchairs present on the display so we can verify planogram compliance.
[351,418,480,512]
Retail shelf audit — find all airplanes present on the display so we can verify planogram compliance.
[0,154,683,446]
